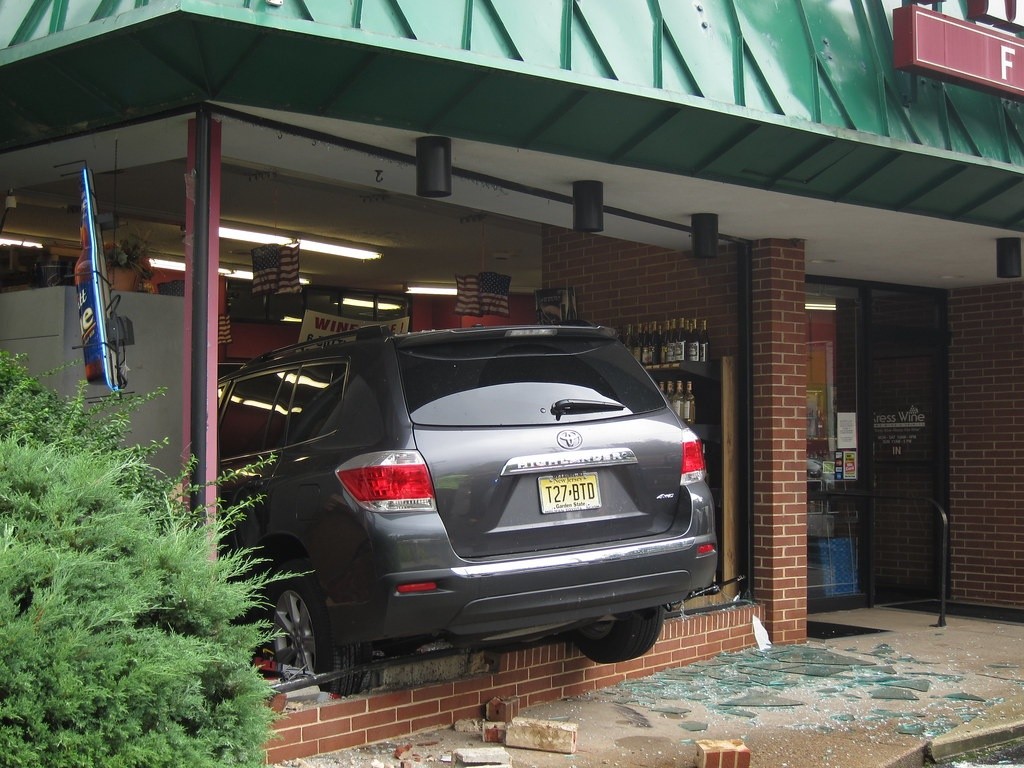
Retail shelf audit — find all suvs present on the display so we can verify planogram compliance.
[217,316,717,696]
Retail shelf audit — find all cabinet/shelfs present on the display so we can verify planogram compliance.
[644,354,742,609]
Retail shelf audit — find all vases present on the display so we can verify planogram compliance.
[108,266,138,291]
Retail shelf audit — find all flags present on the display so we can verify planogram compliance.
[454,272,511,317]
[252,246,302,293]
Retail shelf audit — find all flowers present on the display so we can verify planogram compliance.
[104,224,167,281]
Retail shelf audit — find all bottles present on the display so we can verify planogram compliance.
[618,318,710,424]
[72,170,109,386]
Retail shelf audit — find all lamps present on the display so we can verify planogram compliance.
[219,220,385,260]
[691,213,720,259]
[416,135,453,198]
[330,296,403,310]
[218,262,312,286]
[15,189,186,225]
[996,237,1022,278]
[572,180,604,233]
[148,252,185,272]
[804,303,836,310]
[0,232,44,249]
[402,282,458,296]
